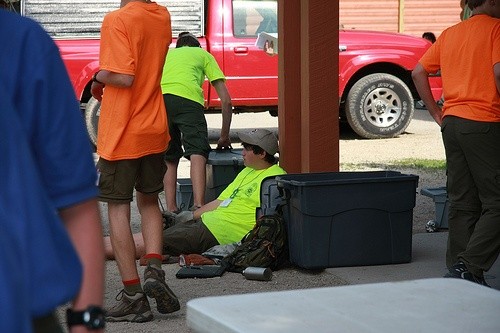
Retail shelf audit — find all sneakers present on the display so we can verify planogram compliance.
[445,263,491,288]
[106,292,152,322]
[144,265,181,315]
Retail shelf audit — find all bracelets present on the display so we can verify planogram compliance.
[93,70,102,83]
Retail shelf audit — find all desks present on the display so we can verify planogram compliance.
[184,278,500,333]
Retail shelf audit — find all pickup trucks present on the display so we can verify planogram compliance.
[49,0,445,140]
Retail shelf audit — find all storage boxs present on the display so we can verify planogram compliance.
[275,171,419,269]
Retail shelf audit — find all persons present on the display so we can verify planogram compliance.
[104,129,289,260]
[91,0,181,322]
[412,0,500,288]
[160,32,233,215]
[0,0,107,333]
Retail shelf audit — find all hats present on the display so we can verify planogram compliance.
[234,127,279,156]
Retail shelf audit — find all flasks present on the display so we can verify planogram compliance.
[242,266,273,281]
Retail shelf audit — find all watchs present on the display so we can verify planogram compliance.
[66,305,106,330]
